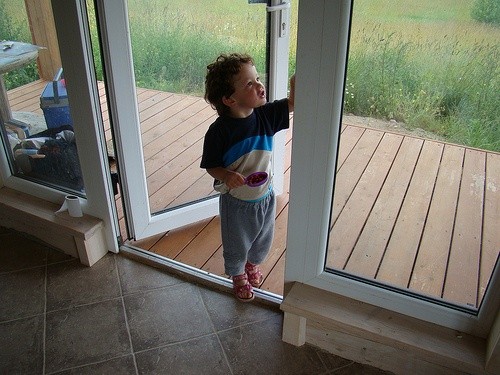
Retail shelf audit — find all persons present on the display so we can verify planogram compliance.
[200,53,295,302]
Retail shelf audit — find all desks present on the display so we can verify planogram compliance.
[0,41,48,138]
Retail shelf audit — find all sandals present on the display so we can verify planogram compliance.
[245,262,263,286]
[233,274,254,301]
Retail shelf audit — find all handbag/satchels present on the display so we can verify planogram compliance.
[13,124,74,179]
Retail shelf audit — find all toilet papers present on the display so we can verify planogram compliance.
[55,194,83,217]
